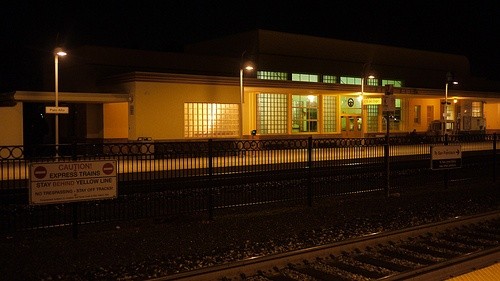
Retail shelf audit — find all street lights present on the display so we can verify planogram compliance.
[361,74,375,142]
[51,47,67,160]
[240,64,254,154]
[445,80,458,144]
